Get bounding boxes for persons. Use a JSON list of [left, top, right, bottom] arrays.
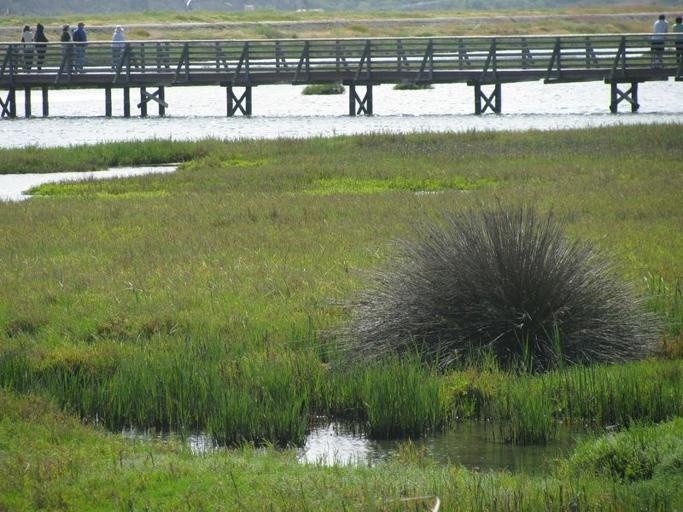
[[673, 16, 683, 67], [112, 25, 124, 69], [651, 15, 666, 69], [22, 24, 34, 71], [61, 24, 73, 72], [72, 23, 88, 72], [34, 23, 48, 71]]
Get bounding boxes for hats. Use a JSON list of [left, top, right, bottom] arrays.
[[24, 25, 30, 31], [114, 25, 124, 31], [62, 22, 86, 30], [37, 23, 44, 31]]
